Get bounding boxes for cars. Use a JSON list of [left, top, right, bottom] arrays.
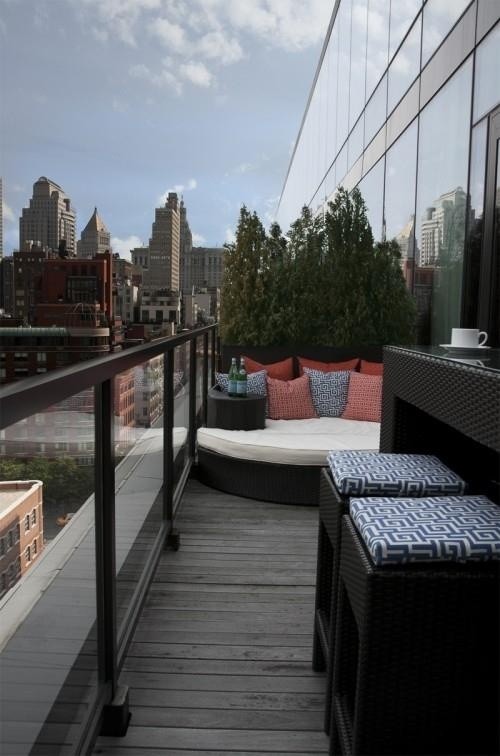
[[55, 510, 76, 526]]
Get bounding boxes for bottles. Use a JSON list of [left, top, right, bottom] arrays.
[[237, 356, 248, 397], [228, 357, 238, 396]]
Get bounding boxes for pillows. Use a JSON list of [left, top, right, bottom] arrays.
[[239, 355, 293, 382], [302, 367, 350, 417], [215, 370, 269, 417], [360, 360, 384, 375], [340, 371, 383, 422], [297, 357, 359, 378], [266, 374, 320, 419]]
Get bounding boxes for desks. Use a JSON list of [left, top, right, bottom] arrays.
[[207, 389, 266, 430], [380, 344, 500, 453]]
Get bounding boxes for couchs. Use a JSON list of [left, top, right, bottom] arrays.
[[194, 345, 383, 505]]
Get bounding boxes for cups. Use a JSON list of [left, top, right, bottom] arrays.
[[463, 359, 485, 367], [451, 327, 489, 346]]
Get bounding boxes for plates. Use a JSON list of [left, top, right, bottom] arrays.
[[441, 353, 492, 362], [438, 343, 492, 352]]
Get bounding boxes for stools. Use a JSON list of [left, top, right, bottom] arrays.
[[330, 494, 500, 756], [312, 449, 465, 735]]
